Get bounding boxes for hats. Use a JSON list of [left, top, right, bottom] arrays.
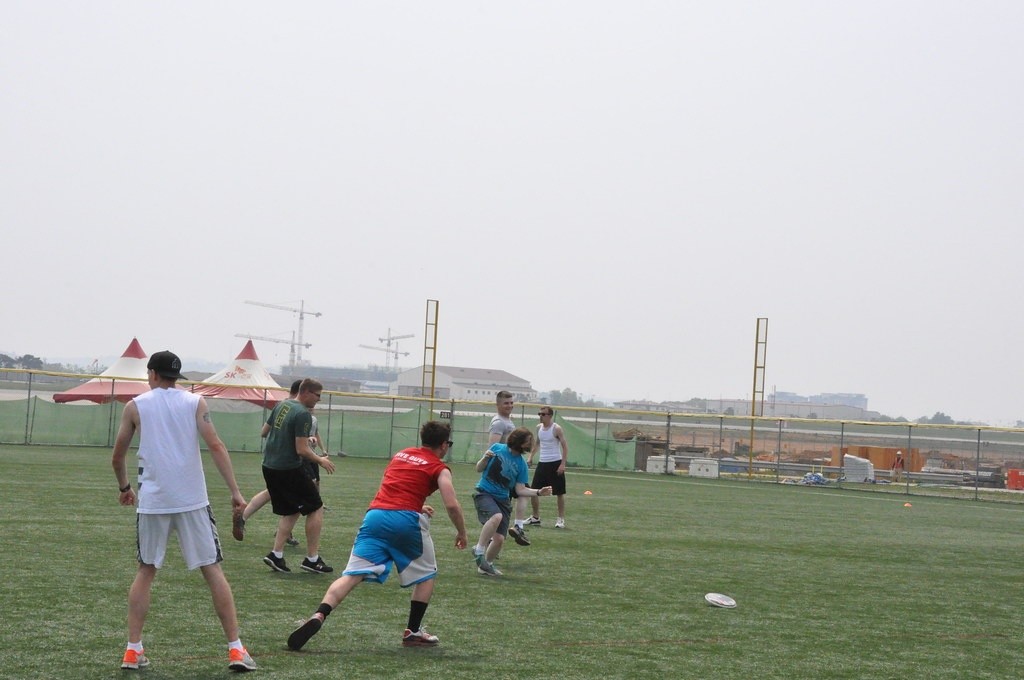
[[147, 351, 188, 381]]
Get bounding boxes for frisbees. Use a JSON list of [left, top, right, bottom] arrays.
[[705, 592, 737, 609]]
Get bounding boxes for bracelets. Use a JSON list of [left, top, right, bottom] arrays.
[[119, 482, 131, 492], [537, 489, 540, 496]]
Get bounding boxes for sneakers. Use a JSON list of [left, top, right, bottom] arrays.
[[555, 517, 564, 528], [228, 646, 256, 670], [478, 560, 503, 576], [287, 611, 324, 651], [300, 558, 333, 575], [471, 545, 486, 567], [509, 522, 530, 546], [523, 515, 540, 526], [487, 538, 500, 559], [121, 648, 149, 669], [273, 529, 300, 545], [402, 625, 439, 647], [263, 552, 291, 574], [233, 508, 246, 541]]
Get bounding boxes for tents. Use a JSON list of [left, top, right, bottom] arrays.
[[54, 337, 186, 403], [187, 340, 291, 411]]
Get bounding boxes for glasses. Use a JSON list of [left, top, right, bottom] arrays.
[[308, 391, 320, 398], [440, 440, 453, 447], [538, 413, 551, 416]]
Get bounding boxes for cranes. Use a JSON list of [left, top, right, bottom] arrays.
[[377, 326, 414, 376], [234, 329, 312, 375], [358, 341, 410, 381], [244, 298, 322, 366]]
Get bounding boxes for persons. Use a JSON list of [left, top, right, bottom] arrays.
[[523, 406, 568, 528], [112, 350, 257, 669], [892, 451, 905, 483], [310, 408, 331, 512], [233, 380, 299, 545], [472, 427, 554, 576], [288, 419, 467, 649], [488, 391, 532, 559], [261, 377, 336, 573]]
